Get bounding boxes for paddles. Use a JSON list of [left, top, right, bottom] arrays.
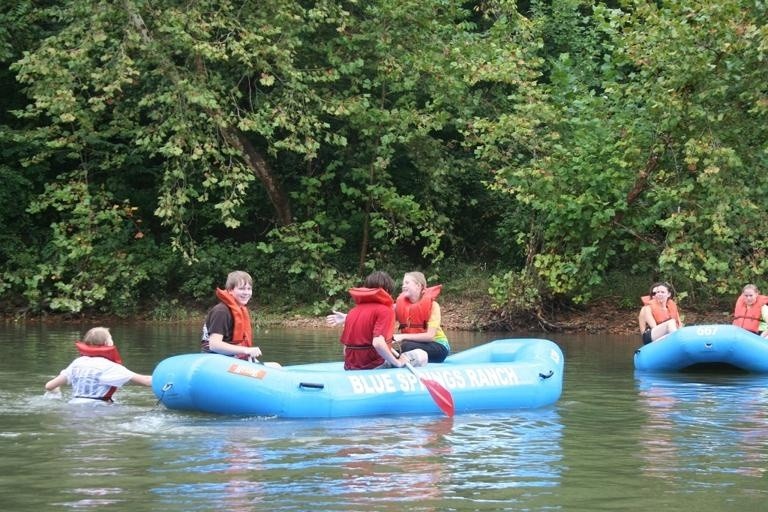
[[388, 347, 454, 420]]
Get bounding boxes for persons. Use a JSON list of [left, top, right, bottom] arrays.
[[45, 326, 153, 405], [642, 284, 681, 345], [732, 283, 768, 339], [328, 270, 450, 365], [638, 283, 659, 336], [339, 270, 429, 369], [200, 269, 282, 370]]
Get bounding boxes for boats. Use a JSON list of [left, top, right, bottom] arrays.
[[148, 337, 565, 423], [629, 321, 766, 378]]
